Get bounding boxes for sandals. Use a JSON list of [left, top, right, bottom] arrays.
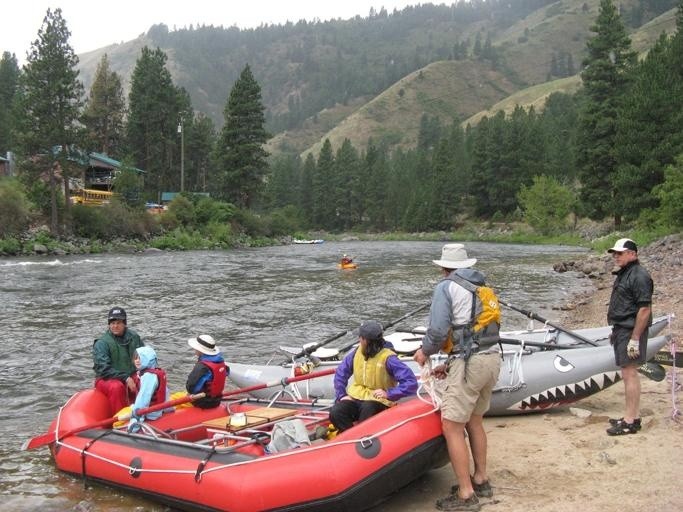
[[609, 417, 641, 427], [606, 424, 638, 436]]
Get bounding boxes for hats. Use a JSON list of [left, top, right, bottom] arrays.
[[188, 334, 221, 357], [107, 306, 126, 322], [608, 238, 640, 255], [433, 242, 478, 269], [358, 319, 383, 339]]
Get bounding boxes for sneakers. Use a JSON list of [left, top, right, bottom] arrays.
[[435, 493, 481, 512], [451, 475, 493, 499]]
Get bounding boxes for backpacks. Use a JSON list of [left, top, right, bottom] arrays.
[[269, 418, 311, 447], [470, 286, 504, 344]]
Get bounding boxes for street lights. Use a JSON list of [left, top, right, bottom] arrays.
[[176, 116, 186, 193]]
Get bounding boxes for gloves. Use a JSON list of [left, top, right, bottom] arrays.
[[626, 339, 640, 360]]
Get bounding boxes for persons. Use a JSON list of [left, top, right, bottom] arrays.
[[111, 346, 168, 436], [413, 243, 502, 510], [170, 334, 230, 407], [92, 307, 141, 417], [606, 237, 653, 436], [328, 322, 418, 435]]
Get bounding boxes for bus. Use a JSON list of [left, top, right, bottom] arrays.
[[72, 189, 116, 205]]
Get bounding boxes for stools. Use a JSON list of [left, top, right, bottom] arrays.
[[242, 406, 299, 421], [203, 412, 268, 438]]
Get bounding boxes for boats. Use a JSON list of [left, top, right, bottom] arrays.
[[46, 383, 456, 508], [383, 310, 674, 354], [241, 335, 674, 415], [295, 240, 315, 245], [337, 263, 356, 270], [314, 239, 324, 244]]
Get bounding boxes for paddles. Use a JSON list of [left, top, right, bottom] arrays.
[[19, 368, 206, 452], [498, 334, 683, 369], [498, 298, 666, 382]]
